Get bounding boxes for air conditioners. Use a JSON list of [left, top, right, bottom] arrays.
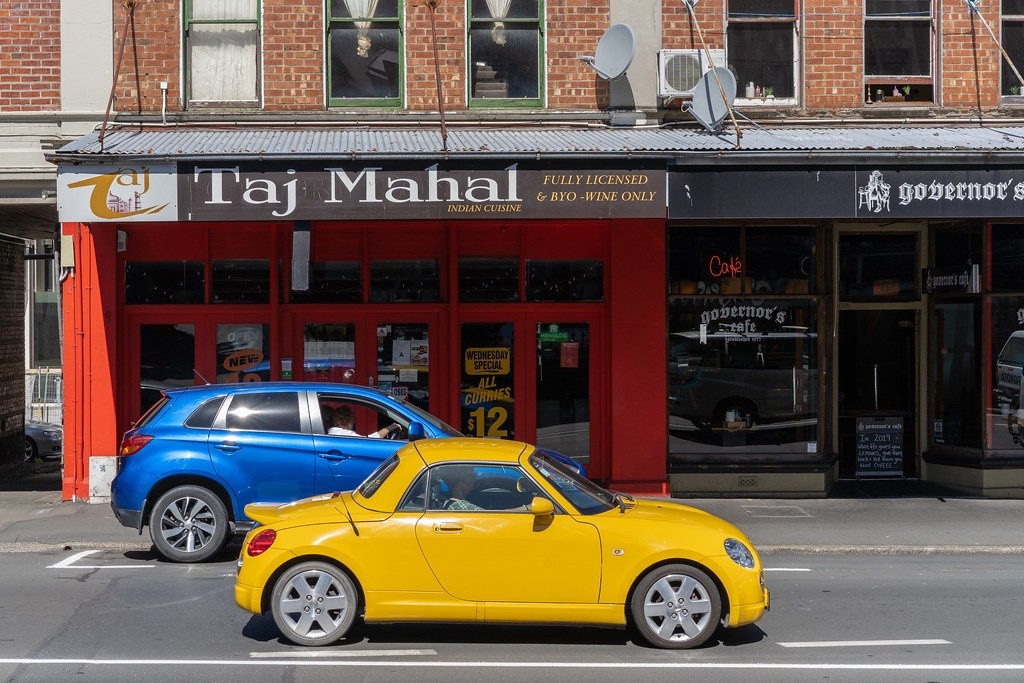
[[656, 48, 728, 107]]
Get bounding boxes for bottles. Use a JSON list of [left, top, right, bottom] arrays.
[[746, 414, 752, 428]]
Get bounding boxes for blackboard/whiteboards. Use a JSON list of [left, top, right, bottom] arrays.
[[855, 417, 904, 478]]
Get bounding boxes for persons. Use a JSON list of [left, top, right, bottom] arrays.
[[320, 404, 335, 435], [438, 464, 544, 512], [328, 404, 403, 439]]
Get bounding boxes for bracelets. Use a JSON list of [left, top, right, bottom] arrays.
[[523, 504, 529, 512]]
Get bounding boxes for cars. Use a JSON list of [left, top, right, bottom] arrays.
[[109, 382, 472, 563], [236, 437, 769, 648]]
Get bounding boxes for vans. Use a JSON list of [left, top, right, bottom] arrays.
[[672, 328, 822, 410], [995, 330, 1024, 421]]
